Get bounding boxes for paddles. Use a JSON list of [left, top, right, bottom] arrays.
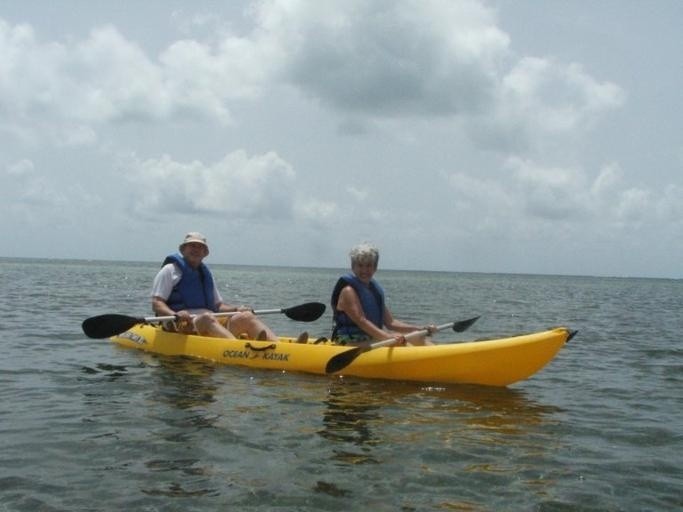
[[80, 302, 326, 339], [326, 315, 480, 374]]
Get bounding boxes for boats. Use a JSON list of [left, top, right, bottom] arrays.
[[107, 314, 576, 392]]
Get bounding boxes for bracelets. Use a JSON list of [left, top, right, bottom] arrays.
[[232, 306, 238, 312]]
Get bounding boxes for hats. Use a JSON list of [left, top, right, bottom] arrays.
[[180, 232, 209, 256]]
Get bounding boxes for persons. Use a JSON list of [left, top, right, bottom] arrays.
[[149, 231, 309, 346], [327, 243, 439, 349]]
